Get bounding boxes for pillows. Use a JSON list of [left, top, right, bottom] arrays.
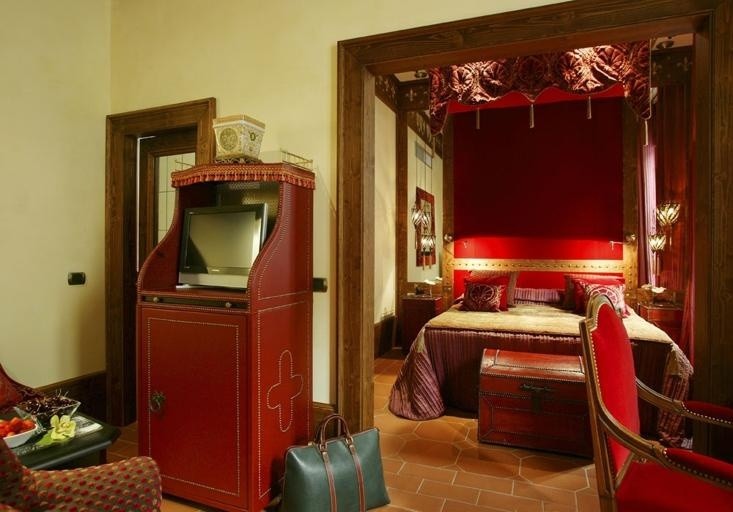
[[457, 269, 518, 313], [562, 272, 631, 318]]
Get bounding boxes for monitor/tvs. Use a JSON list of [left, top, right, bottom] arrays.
[[178, 203, 268, 290]]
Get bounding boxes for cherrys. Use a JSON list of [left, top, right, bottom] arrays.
[[17, 388, 78, 429]]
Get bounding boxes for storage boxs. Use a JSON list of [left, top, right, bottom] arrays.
[[477, 347, 594, 460]]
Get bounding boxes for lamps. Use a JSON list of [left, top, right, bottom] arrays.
[[648, 40, 681, 253], [411, 71, 436, 271], [443, 233, 456, 242], [610, 233, 636, 245]]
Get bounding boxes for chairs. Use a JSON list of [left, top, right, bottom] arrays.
[[0, 363, 46, 415], [579, 294, 733, 512], [0, 435, 163, 511]]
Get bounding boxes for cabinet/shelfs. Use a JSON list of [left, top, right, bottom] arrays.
[[136, 162, 315, 512]]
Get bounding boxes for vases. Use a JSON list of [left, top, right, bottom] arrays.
[[212, 116, 266, 164]]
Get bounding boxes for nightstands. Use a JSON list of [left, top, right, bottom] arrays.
[[401, 295, 444, 355], [636, 299, 683, 344]]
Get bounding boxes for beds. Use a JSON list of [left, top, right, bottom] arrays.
[[388, 288, 695, 451]]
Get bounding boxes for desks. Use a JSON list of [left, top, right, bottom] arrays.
[[0, 408, 121, 471]]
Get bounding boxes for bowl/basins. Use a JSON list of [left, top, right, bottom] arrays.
[[14, 396, 81, 431], [1, 423, 38, 450]]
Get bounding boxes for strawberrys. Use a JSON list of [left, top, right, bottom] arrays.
[[0, 416, 36, 438]]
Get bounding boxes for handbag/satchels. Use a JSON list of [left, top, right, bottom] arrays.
[[280, 411, 393, 512]]
